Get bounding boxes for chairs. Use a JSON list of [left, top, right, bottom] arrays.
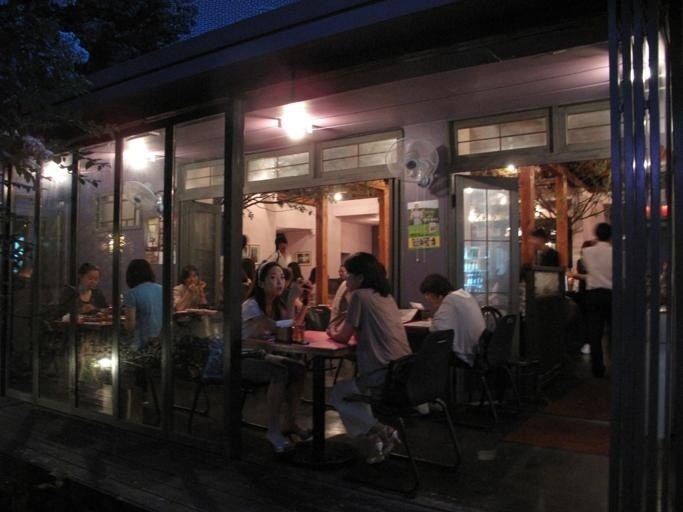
[[38, 290, 228, 421], [341, 328, 462, 484], [440, 313, 520, 426], [464, 307, 519, 415], [241, 374, 334, 440], [303, 305, 367, 390]]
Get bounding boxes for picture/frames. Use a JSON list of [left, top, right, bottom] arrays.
[[140, 215, 162, 251], [294, 250, 312, 267], [245, 242, 264, 266]]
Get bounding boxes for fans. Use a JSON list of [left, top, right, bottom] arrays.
[[382, 136, 443, 191], [120, 178, 167, 225]]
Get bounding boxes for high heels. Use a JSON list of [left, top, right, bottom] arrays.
[[266, 429, 292, 452], [280, 425, 314, 442], [366, 425, 400, 464]]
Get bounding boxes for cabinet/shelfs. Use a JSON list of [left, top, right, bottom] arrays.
[[522, 260, 574, 406]]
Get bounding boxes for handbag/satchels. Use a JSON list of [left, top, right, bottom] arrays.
[[305, 308, 330, 331]]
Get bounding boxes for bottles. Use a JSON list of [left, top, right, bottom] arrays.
[[463, 246, 506, 287]]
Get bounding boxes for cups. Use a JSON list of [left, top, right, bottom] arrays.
[[292, 322, 305, 342], [276, 326, 291, 343]]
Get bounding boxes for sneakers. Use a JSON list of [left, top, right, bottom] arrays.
[[430, 398, 446, 411], [413, 402, 429, 414]]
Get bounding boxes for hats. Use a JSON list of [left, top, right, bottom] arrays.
[[275, 233, 287, 245]]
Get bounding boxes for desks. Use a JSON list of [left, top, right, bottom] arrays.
[[243, 329, 362, 473], [403, 321, 437, 354]]
